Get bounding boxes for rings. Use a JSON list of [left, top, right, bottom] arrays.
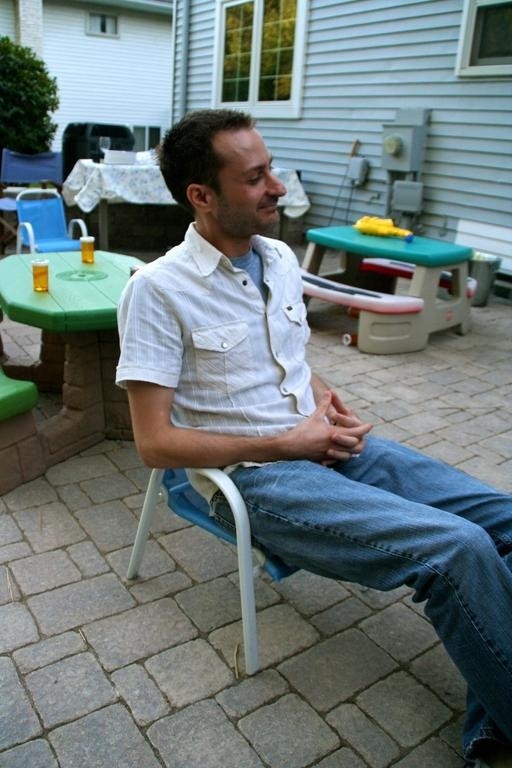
[[350, 453, 361, 460]]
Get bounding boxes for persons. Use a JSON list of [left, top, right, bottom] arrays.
[[115, 110, 512, 768]]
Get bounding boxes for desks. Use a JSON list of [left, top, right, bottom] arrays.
[[0, 251, 149, 460], [301, 225, 478, 351], [60, 156, 312, 255]]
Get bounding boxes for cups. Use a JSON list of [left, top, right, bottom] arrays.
[[32, 260, 48, 292], [80, 236, 95, 264]]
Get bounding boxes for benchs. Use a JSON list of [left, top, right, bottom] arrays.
[[299, 268, 423, 352], [363, 258, 479, 324]]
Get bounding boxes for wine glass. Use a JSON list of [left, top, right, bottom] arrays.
[[99, 136, 111, 162]]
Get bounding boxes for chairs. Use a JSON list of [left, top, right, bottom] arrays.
[[0, 149, 65, 250], [128, 450, 306, 680], [16, 188, 87, 256]]
